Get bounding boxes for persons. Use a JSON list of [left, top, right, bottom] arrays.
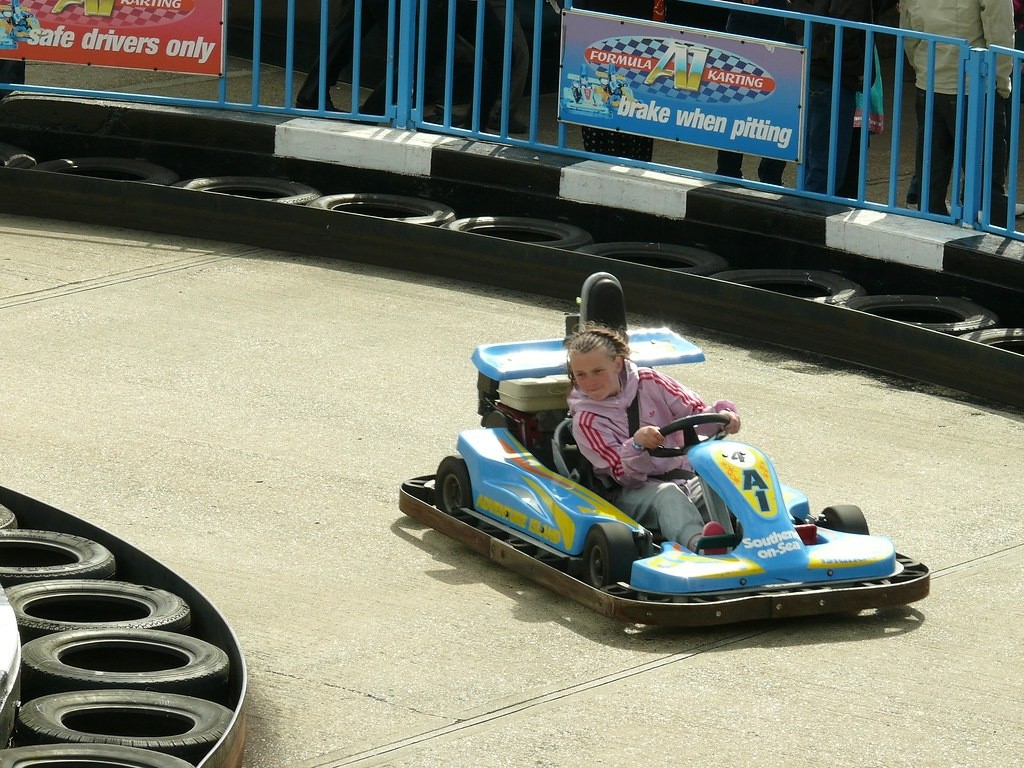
[[708, 0, 797, 186], [295, 0, 529, 135], [899, 0, 1024, 228], [581, 0, 654, 162], [784, 0, 915, 199], [567, 325, 740, 555]]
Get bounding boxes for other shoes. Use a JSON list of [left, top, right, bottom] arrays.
[[702, 522, 727, 556], [296, 75, 351, 121], [1014, 203, 1024, 216], [434, 105, 468, 127], [488, 114, 527, 134], [906, 199, 950, 211], [946, 205, 983, 228]]
